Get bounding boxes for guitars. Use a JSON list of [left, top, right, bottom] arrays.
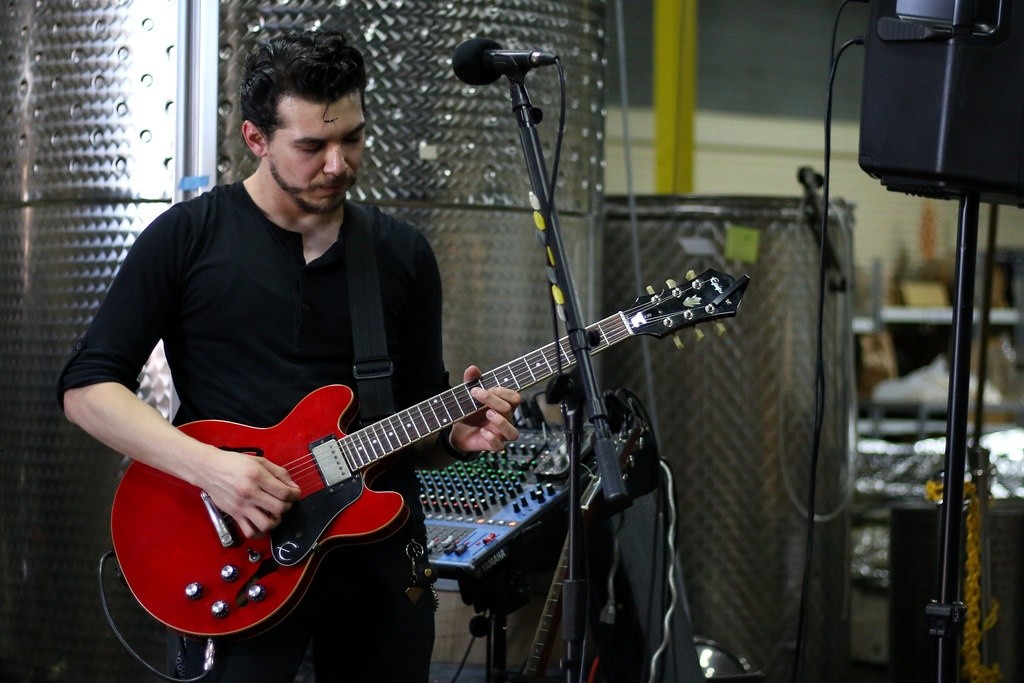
[[111, 266, 750, 644]]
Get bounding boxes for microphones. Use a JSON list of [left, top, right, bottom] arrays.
[[453, 39, 560, 86]]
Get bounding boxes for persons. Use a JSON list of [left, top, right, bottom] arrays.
[[57, 32, 522, 682]]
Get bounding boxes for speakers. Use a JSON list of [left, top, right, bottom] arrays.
[[858, 2, 1023, 204]]
